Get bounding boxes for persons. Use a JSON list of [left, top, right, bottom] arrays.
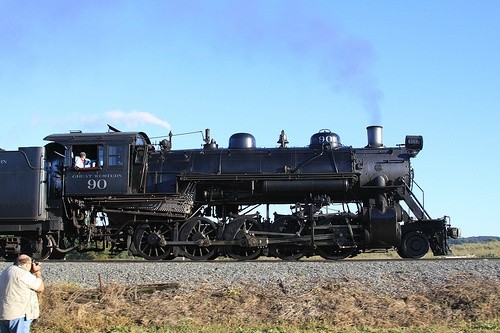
[[0, 254, 44, 333], [75, 152, 86, 168]]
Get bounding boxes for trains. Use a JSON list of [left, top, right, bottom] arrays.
[[0, 125, 461, 262]]
